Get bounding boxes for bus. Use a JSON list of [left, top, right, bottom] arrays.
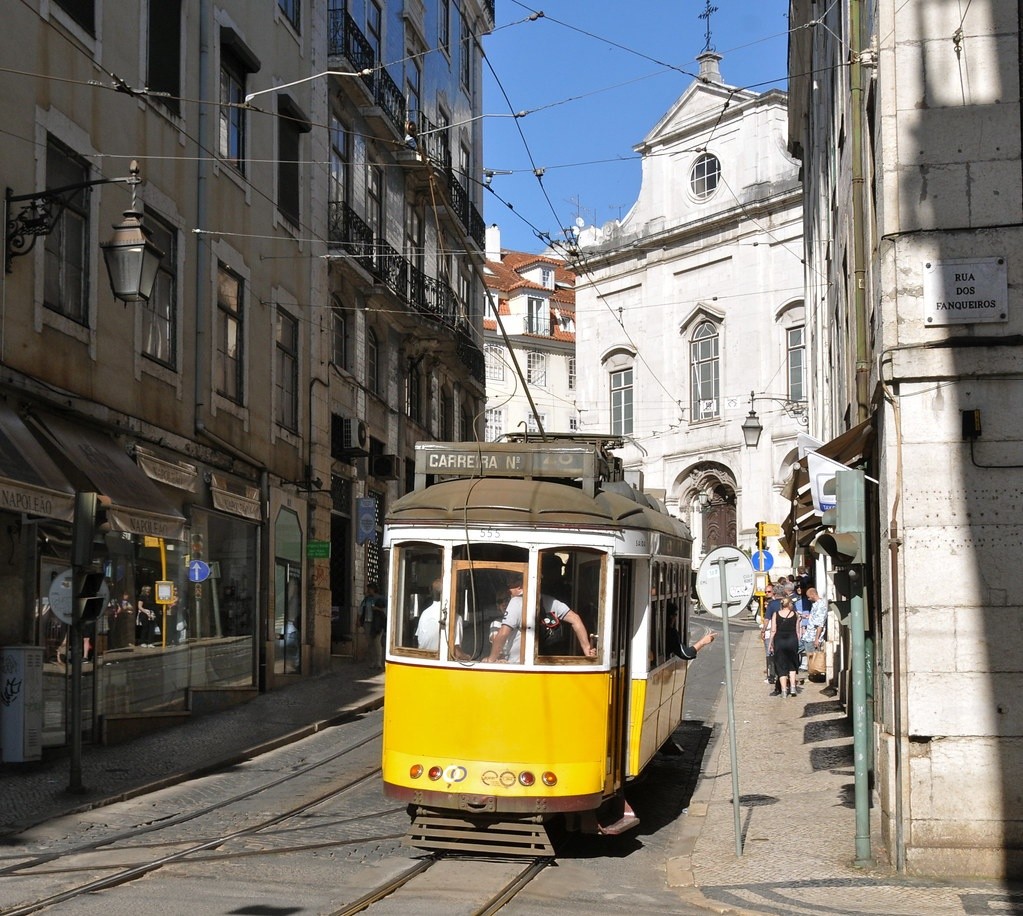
[[380, 120, 696, 859]]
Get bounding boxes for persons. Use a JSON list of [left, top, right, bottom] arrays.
[[414, 551, 597, 662], [357, 583, 387, 668], [293, 613, 301, 668], [666, 603, 718, 660], [53, 623, 94, 664], [754, 566, 827, 698], [108, 582, 189, 649]]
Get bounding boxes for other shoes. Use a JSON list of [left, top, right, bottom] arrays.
[[781, 691, 789, 699], [148, 644, 155, 648], [140, 643, 147, 648], [764, 679, 768, 683], [791, 687, 798, 696]]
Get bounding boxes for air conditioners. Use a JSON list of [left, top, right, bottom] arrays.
[[342, 419, 372, 452], [375, 454, 401, 481]]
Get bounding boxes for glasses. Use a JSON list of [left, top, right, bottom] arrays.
[[670, 613, 677, 617], [765, 591, 771, 593], [497, 596, 510, 604]]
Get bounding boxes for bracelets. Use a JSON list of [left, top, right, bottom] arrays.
[[761, 631, 765, 633]]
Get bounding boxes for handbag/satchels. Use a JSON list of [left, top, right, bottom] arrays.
[[766, 652, 778, 684], [808, 648, 826, 675]]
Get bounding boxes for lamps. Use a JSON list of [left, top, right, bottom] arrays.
[[741, 390, 808, 449], [4, 159, 165, 308]]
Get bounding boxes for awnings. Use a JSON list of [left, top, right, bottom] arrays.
[[0, 397, 76, 522], [776, 416, 873, 569], [126, 443, 198, 495], [23, 401, 188, 543], [205, 472, 262, 521]]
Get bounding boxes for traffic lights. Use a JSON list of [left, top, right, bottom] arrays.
[[821, 471, 864, 527], [79, 597, 105, 622], [76, 570, 106, 596], [755, 521, 767, 549], [68, 491, 113, 567], [814, 532, 867, 566]]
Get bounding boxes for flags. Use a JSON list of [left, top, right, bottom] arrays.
[[806, 452, 851, 516]]
